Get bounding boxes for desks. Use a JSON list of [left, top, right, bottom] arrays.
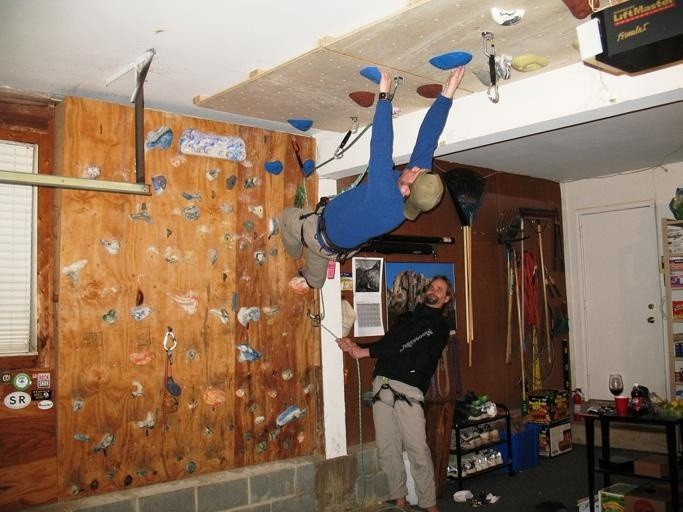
[[579, 399, 683, 512]]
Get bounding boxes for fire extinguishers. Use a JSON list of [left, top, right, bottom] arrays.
[[571, 388, 582, 421]]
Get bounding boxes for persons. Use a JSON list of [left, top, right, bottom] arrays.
[[278, 65, 466, 287], [333, 276, 452, 512]]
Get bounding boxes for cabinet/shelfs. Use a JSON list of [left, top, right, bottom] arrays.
[[447, 403, 514, 491], [661, 218, 683, 401]]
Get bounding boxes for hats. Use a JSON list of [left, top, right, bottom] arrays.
[[403, 170, 445, 222]]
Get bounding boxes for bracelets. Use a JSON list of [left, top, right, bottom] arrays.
[[379, 91, 395, 102]]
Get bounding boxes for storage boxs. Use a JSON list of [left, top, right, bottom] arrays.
[[528, 388, 572, 457], [598, 483, 639, 512], [624, 480, 673, 512]]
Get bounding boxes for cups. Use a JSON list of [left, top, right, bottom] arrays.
[[609, 375, 629, 417]]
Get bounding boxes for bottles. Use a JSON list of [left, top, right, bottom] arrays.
[[631, 383, 643, 417]]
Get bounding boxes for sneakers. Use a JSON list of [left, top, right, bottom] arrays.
[[447, 399, 504, 480]]
